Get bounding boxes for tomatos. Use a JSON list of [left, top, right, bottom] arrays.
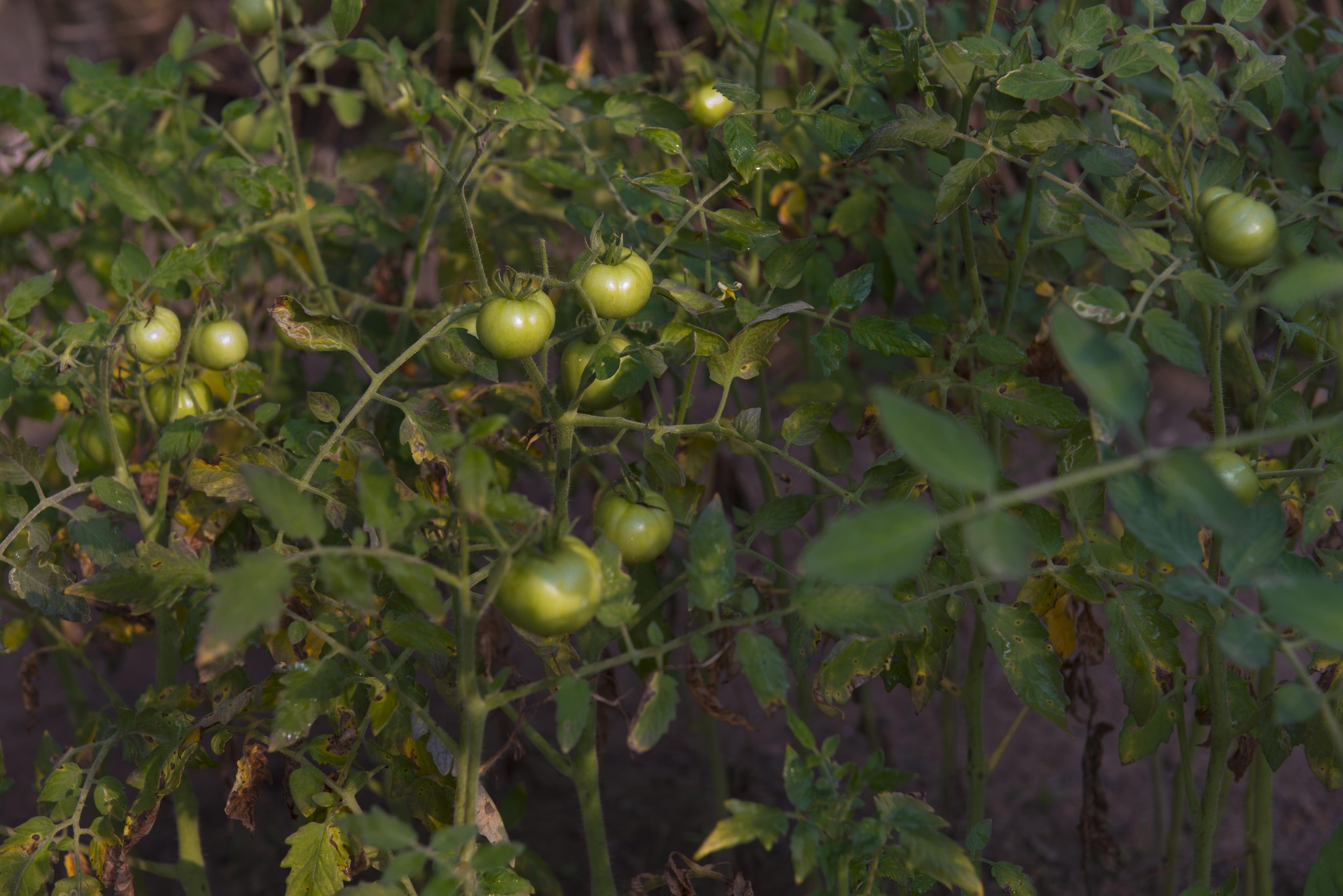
[[0, 83, 1277, 634]]
